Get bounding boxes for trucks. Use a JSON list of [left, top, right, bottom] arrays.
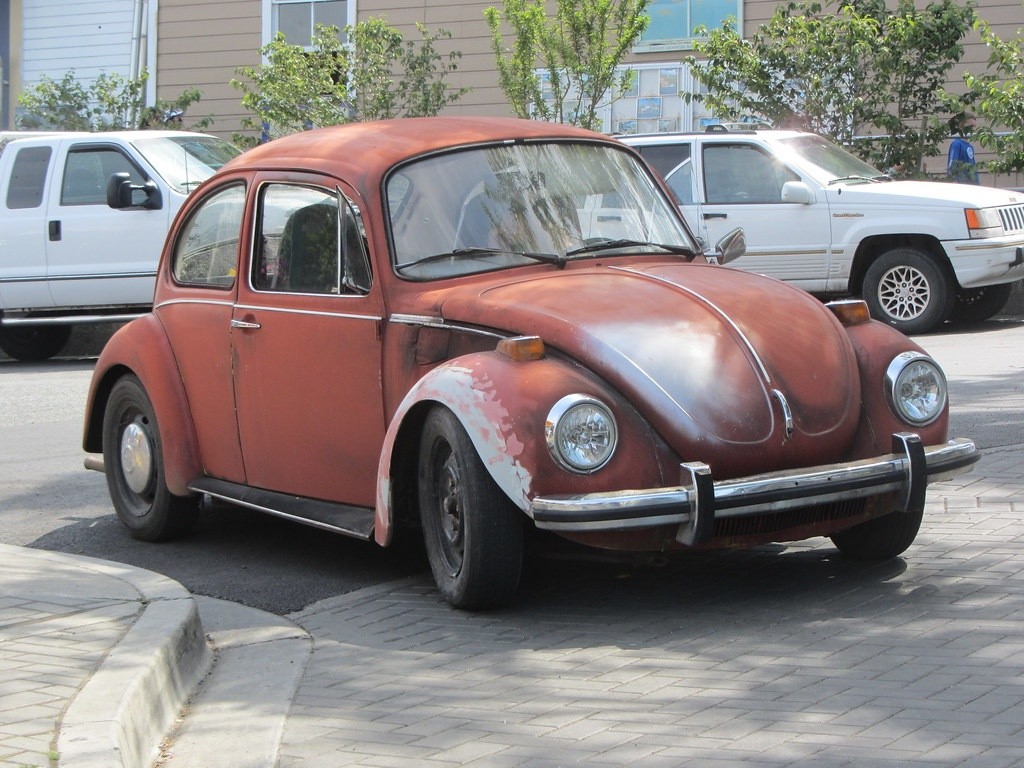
[[0, 130, 344, 364]]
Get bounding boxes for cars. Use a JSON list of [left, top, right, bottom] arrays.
[[81, 113, 983, 610]]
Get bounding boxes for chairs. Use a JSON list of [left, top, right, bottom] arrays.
[[69, 169, 100, 198], [457, 191, 523, 249], [270, 204, 370, 294]]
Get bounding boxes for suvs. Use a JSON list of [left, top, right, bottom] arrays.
[[570, 128, 1024, 334]]
